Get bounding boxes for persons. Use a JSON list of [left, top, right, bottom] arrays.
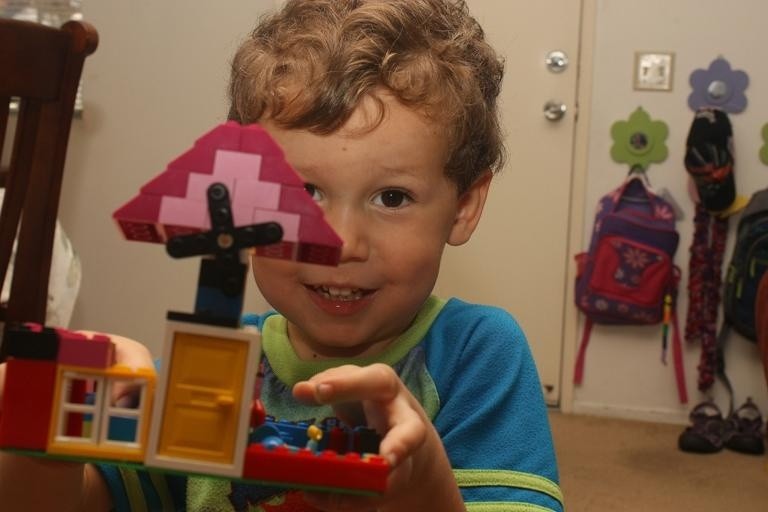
[[1, 0, 566, 512]]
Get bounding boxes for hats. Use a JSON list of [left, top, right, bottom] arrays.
[[685, 108, 736, 210]]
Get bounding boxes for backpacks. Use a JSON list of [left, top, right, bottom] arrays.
[[715, 184, 768, 399], [574, 162, 687, 406]]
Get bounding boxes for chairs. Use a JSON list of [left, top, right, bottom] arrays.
[[0, 18, 107, 332]]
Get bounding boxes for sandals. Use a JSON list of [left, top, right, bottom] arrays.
[[677, 399, 766, 454]]
